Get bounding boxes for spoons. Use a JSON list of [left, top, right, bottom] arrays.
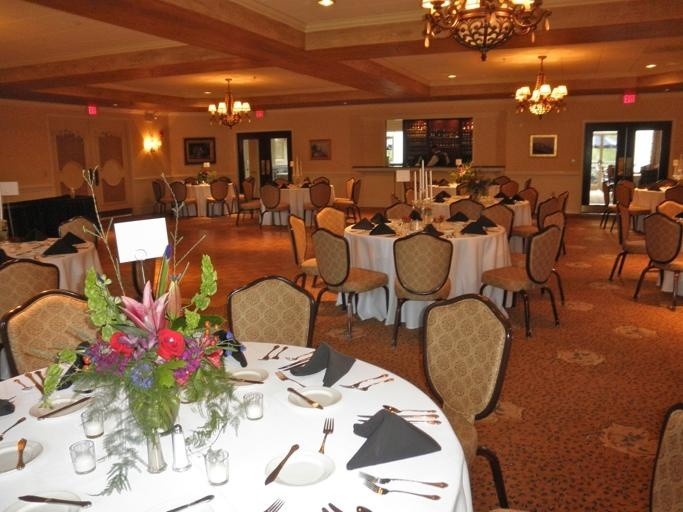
[[356, 505, 372, 511], [17, 438, 26, 469], [383, 405, 438, 413], [12, 379, 30, 390]]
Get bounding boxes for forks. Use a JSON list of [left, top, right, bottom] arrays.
[[259, 345, 288, 360], [274, 370, 304, 387], [359, 473, 446, 500], [286, 351, 314, 361], [339, 374, 393, 391], [317, 417, 334, 453], [263, 498, 286, 512]]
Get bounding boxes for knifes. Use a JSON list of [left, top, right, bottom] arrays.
[[166, 494, 214, 511], [357, 414, 441, 425], [263, 444, 299, 485], [17, 495, 91, 507], [321, 502, 344, 512], [0, 416, 25, 441], [286, 388, 324, 410], [24, 370, 46, 394], [227, 377, 265, 384]]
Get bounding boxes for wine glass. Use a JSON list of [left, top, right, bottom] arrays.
[[455, 159, 461, 168]]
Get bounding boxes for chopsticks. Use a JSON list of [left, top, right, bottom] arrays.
[[277, 357, 309, 370], [37, 397, 91, 419]]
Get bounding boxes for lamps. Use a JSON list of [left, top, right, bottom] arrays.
[[420, 0, 552, 61], [142, 129, 162, 153], [514, 56, 569, 120], [207, 78, 253, 130]]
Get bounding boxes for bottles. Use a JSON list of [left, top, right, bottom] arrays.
[[172, 424, 191, 471]]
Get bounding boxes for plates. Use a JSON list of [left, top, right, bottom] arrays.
[[0, 439, 43, 474], [0, 399, 14, 416], [226, 368, 269, 386], [349, 212, 501, 238], [29, 398, 85, 419], [0, 491, 82, 512], [70, 243, 90, 249], [287, 385, 342, 408], [0, 238, 61, 259], [265, 451, 333, 485]]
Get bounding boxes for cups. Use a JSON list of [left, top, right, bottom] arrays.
[[204, 450, 229, 485], [68, 440, 96, 475], [242, 391, 263, 420], [80, 408, 103, 437]]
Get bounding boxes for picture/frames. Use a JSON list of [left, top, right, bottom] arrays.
[[184, 137, 216, 165], [529, 134, 558, 158], [309, 139, 331, 161]]
